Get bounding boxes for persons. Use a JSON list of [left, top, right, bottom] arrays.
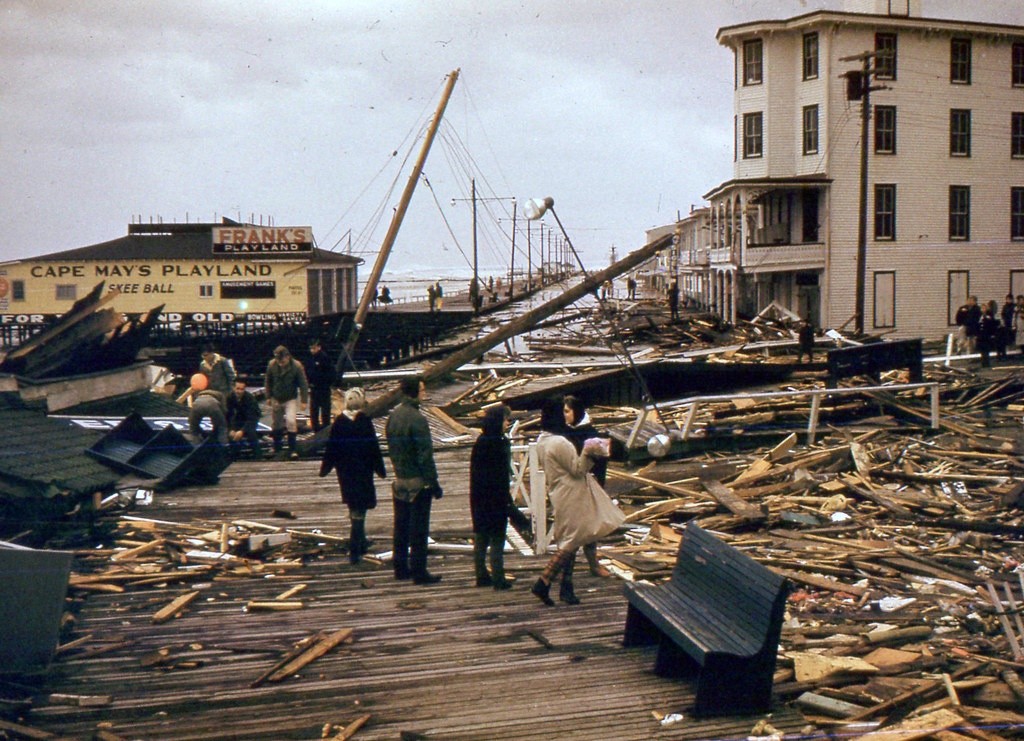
[[427, 282, 443, 312], [266, 345, 308, 460], [469, 406, 512, 589], [371, 285, 393, 311], [667, 283, 679, 321], [489, 276, 502, 294], [602, 279, 614, 300], [467, 279, 479, 302], [956, 294, 1024, 368], [626, 277, 636, 300], [531, 394, 627, 607], [189, 342, 264, 463], [385, 376, 443, 585], [795, 319, 814, 365], [305, 339, 336, 433], [318, 388, 387, 563]]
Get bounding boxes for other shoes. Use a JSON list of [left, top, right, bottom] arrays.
[[559, 589, 580, 604], [476, 576, 493, 587], [494, 580, 512, 590], [590, 565, 610, 578], [531, 577, 555, 606], [414, 574, 442, 585], [396, 571, 412, 580]]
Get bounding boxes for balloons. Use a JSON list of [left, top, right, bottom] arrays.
[[190, 373, 208, 391]]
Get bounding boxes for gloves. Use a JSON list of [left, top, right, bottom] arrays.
[[432, 485, 442, 499]]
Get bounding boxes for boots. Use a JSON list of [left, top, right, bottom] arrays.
[[351, 517, 368, 564], [272, 428, 299, 458]]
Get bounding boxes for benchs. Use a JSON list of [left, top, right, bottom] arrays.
[[85, 413, 234, 493], [489, 291, 498, 303], [814, 337, 925, 389], [623, 523, 796, 719]]
